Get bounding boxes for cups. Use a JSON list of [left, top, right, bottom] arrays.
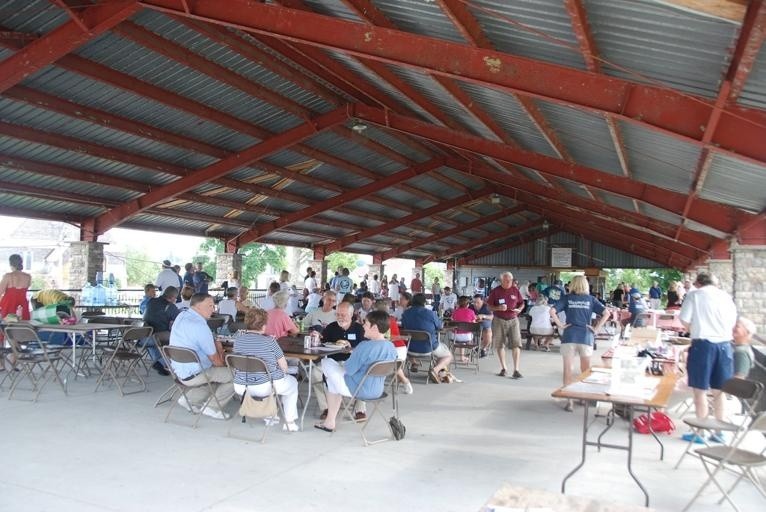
[[312, 337, 321, 347]]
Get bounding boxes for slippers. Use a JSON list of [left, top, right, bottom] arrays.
[[314, 424, 332, 432]]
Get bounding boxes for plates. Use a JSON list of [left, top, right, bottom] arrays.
[[323, 341, 348, 347]]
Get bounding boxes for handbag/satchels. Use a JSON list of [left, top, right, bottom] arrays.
[[238, 390, 276, 418], [633, 412, 675, 433]]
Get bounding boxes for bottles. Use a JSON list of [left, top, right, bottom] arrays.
[[300, 320, 304, 332]]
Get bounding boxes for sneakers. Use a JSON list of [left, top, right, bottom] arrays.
[[355, 412, 366, 422], [512, 372, 521, 378], [282, 423, 298, 432], [403, 378, 412, 395], [319, 409, 327, 419], [499, 370, 508, 376], [428, 369, 440, 384], [682, 433, 724, 444], [152, 360, 169, 375], [177, 395, 229, 419]]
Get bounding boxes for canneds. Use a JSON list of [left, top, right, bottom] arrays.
[[311, 336, 320, 346]]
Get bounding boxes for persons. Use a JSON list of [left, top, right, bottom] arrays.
[[623, 292, 642, 328], [0, 254, 32, 371], [529, 294, 554, 351], [449, 296, 476, 360], [140, 284, 155, 314], [550, 275, 609, 409], [678, 271, 737, 444], [402, 294, 453, 383], [649, 281, 661, 309], [312, 303, 368, 421], [31, 289, 75, 310], [732, 316, 757, 380], [613, 282, 642, 307], [315, 312, 397, 430], [219, 286, 246, 336], [235, 309, 299, 432], [487, 272, 524, 378], [264, 291, 298, 337], [143, 286, 179, 375], [469, 294, 492, 356], [528, 276, 571, 304], [667, 280, 681, 308], [270, 265, 376, 327], [156, 260, 213, 309], [169, 292, 236, 419], [239, 287, 256, 309], [381, 274, 457, 318]]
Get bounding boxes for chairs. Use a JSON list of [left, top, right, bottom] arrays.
[[0, 315, 480, 446], [514, 304, 766, 512]]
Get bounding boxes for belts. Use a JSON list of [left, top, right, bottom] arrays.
[[182, 372, 199, 381]]
